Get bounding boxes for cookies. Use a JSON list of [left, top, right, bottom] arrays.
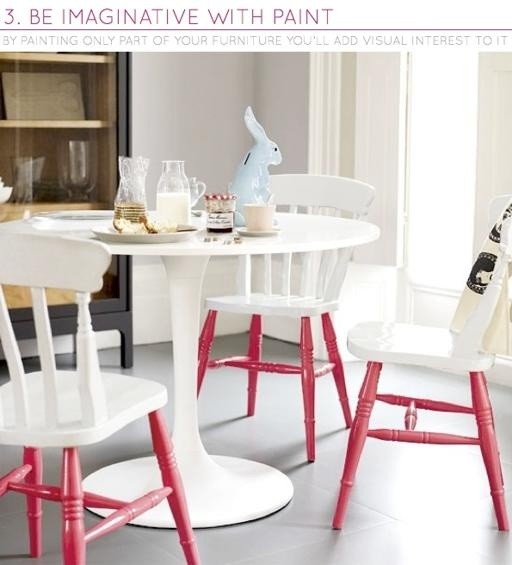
[[113, 204, 147, 233]]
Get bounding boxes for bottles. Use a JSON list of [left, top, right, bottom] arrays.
[[156, 160, 206, 230], [113, 155, 150, 232]]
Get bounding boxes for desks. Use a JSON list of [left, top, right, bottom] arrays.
[[2, 209, 381, 530]]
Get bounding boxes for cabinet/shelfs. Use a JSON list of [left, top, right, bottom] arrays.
[[0, 51, 133, 369]]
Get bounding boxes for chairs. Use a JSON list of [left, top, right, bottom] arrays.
[[0, 227, 200, 564], [190, 172, 510, 534]]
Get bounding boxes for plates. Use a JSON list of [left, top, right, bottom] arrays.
[[237, 230, 278, 237], [92, 223, 205, 244]]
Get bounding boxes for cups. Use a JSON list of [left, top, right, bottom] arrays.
[[244, 205, 276, 232], [10, 156, 46, 203]]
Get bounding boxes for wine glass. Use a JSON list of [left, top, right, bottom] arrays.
[[55, 138, 99, 202]]
